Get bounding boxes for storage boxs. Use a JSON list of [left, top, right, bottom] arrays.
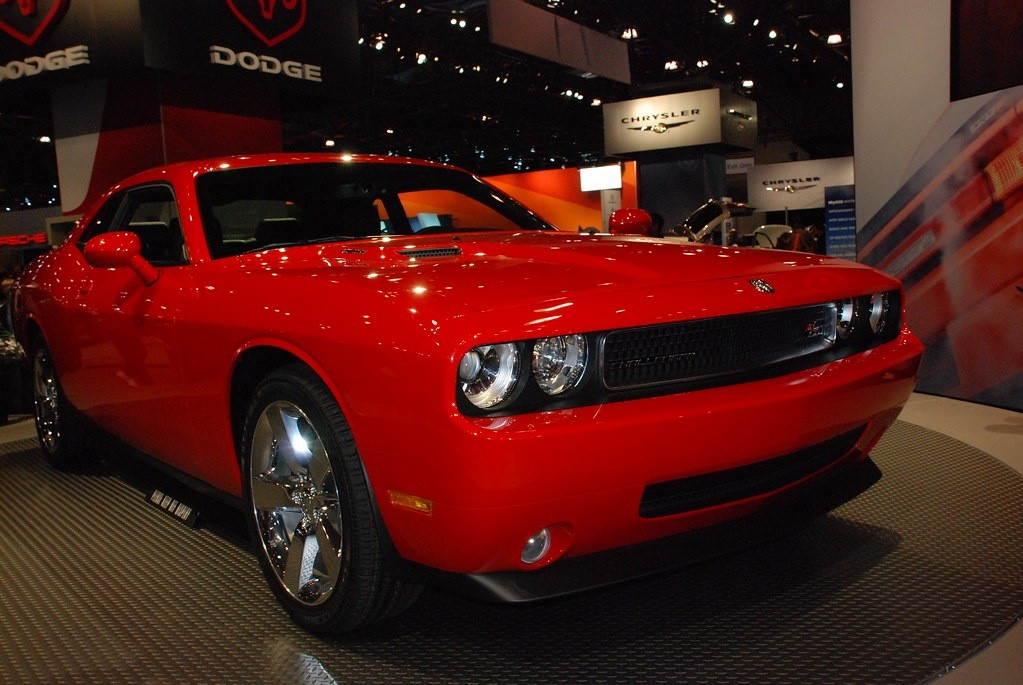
[[45, 214, 82, 245]]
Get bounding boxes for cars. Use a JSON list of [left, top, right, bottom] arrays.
[[660, 194, 824, 254]]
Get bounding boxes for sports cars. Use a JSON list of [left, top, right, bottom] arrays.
[[10, 149, 928, 640]]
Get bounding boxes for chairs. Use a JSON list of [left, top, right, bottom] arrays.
[[168, 216, 225, 262], [128, 221, 168, 235], [254, 218, 297, 238]]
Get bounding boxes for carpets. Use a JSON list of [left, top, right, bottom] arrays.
[[0, 420, 1023, 685]]
